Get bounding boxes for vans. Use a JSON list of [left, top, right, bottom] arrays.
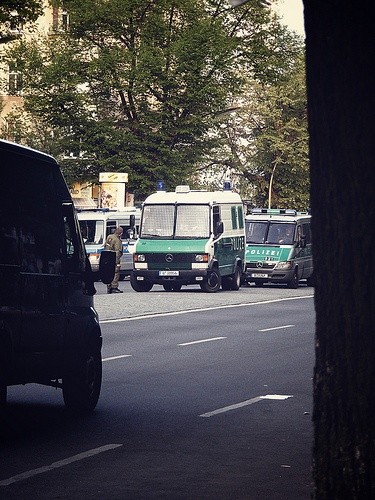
[[240, 209, 314, 290], [130, 180, 246, 294], [73, 206, 142, 283], [0, 137, 104, 415]]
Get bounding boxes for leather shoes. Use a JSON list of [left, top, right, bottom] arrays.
[[106, 287, 123, 293]]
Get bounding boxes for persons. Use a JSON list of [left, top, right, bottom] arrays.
[[104, 227, 124, 294]]
